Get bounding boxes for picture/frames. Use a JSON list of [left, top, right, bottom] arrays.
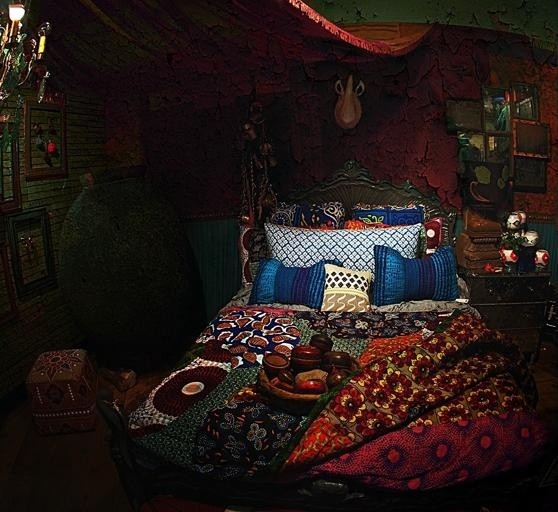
[[0, 97, 67, 325], [445, 82, 551, 194]]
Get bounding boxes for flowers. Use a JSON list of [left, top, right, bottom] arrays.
[[494, 232, 528, 250]]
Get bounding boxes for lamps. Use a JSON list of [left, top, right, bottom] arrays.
[[0, 1, 54, 122]]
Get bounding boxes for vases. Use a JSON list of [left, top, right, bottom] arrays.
[[498, 247, 520, 271]]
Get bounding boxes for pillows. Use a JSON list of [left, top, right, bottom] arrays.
[[248, 213, 460, 314]]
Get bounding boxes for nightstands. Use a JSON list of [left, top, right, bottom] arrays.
[[458, 268, 552, 375]]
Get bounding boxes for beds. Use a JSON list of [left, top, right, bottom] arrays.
[[111, 160, 556, 512]]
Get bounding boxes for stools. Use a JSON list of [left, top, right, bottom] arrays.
[[26, 348, 99, 433]]
